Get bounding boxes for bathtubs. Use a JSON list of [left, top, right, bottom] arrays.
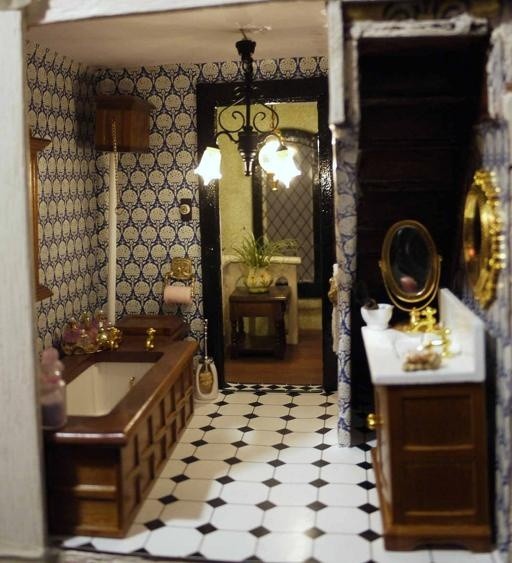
[[26, 335, 198, 539]]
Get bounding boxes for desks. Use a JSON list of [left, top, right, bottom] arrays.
[[228, 285, 293, 359]]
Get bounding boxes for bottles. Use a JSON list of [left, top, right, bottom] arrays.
[[40, 347, 67, 432], [62, 309, 122, 356]]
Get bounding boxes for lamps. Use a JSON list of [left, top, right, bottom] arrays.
[[193, 27, 303, 191]]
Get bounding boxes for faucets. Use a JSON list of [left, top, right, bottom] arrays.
[[145, 327, 157, 350], [417, 322, 451, 356], [409, 307, 437, 333]]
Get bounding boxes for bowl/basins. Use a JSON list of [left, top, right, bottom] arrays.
[[359, 303, 394, 332]]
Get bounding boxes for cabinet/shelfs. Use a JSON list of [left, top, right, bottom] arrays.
[[366, 379, 496, 551]]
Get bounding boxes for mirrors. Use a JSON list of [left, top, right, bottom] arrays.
[[460, 169, 504, 300], [375, 218, 443, 334]]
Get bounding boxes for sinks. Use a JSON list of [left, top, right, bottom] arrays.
[[388, 331, 438, 366]]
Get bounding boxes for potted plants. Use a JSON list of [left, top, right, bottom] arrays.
[[234, 232, 302, 293]]
